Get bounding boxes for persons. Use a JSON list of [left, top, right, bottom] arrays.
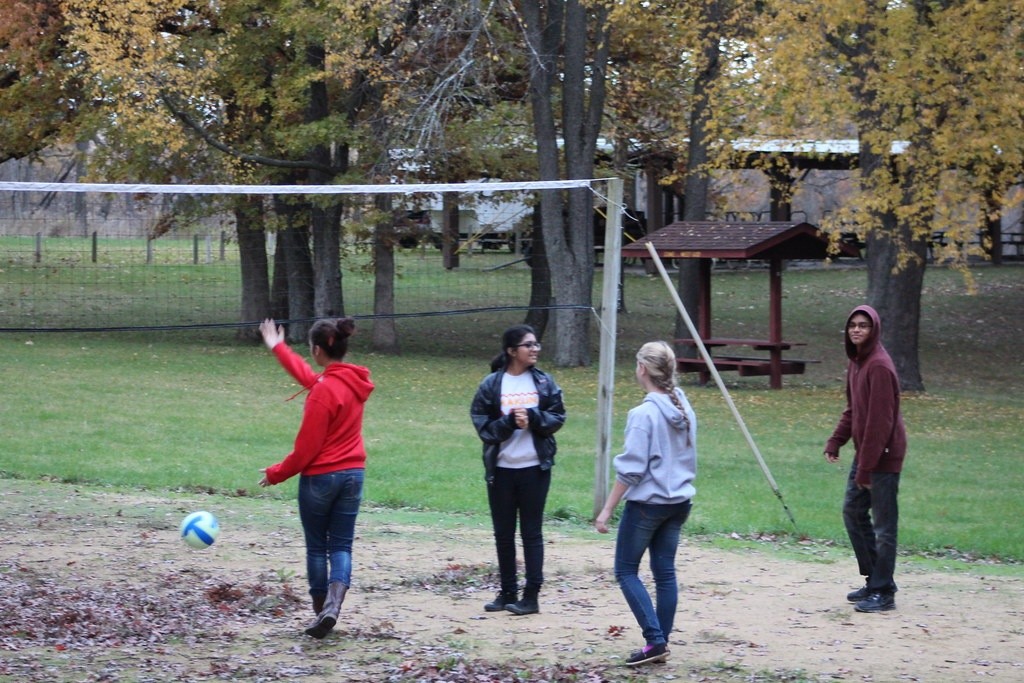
[[469, 324, 566, 615], [595, 341, 697, 665], [822, 304, 907, 612], [258, 317, 375, 639]]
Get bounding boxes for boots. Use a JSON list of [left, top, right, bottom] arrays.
[[305, 582, 348, 639], [310, 592, 327, 619]]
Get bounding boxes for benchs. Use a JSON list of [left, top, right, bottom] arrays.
[[718, 355, 822, 363], [676, 358, 770, 367]]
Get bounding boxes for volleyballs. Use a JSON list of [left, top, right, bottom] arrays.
[[179, 510, 219, 550]]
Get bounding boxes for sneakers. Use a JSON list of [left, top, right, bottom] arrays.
[[503, 594, 539, 615], [855, 590, 895, 613], [847, 585, 873, 601], [485, 590, 517, 611]]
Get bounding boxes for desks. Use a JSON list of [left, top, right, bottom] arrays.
[[671, 337, 809, 389]]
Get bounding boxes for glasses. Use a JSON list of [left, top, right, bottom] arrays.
[[850, 323, 873, 329], [510, 342, 542, 348]]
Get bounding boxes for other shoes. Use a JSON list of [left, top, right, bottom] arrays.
[[625, 643, 670, 666]]
